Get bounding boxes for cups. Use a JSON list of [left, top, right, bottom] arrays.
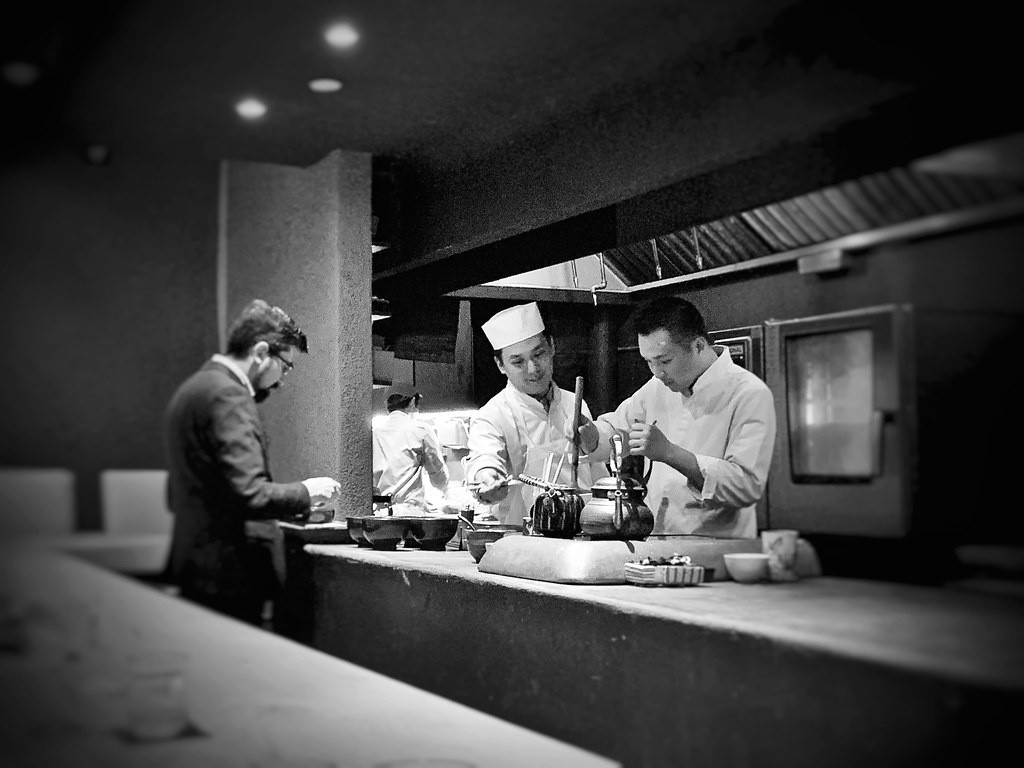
[[761, 529, 798, 582]]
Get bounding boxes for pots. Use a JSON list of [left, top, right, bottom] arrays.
[[467, 521, 524, 531]]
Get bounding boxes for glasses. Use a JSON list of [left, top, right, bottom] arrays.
[[268, 347, 294, 376]]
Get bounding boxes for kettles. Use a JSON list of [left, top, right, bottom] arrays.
[[579, 433, 654, 541]]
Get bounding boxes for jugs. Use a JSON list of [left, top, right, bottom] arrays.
[[518, 473, 585, 534]]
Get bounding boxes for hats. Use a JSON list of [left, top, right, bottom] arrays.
[[480, 301, 544, 351], [387, 382, 422, 409]]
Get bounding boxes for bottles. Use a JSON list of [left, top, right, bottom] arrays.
[[461, 505, 474, 550]]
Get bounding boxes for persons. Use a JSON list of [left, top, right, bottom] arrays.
[[567, 295, 776, 539], [369, 391, 451, 517], [160, 297, 343, 633], [462, 301, 613, 531]]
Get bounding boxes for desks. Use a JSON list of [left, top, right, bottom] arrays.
[[0, 530, 624, 767], [302, 534, 1024, 768]]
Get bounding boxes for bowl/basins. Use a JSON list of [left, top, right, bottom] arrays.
[[465, 530, 506, 563], [723, 553, 771, 580], [345, 515, 383, 548], [361, 516, 410, 550], [399, 516, 437, 548], [405, 519, 459, 551]]
